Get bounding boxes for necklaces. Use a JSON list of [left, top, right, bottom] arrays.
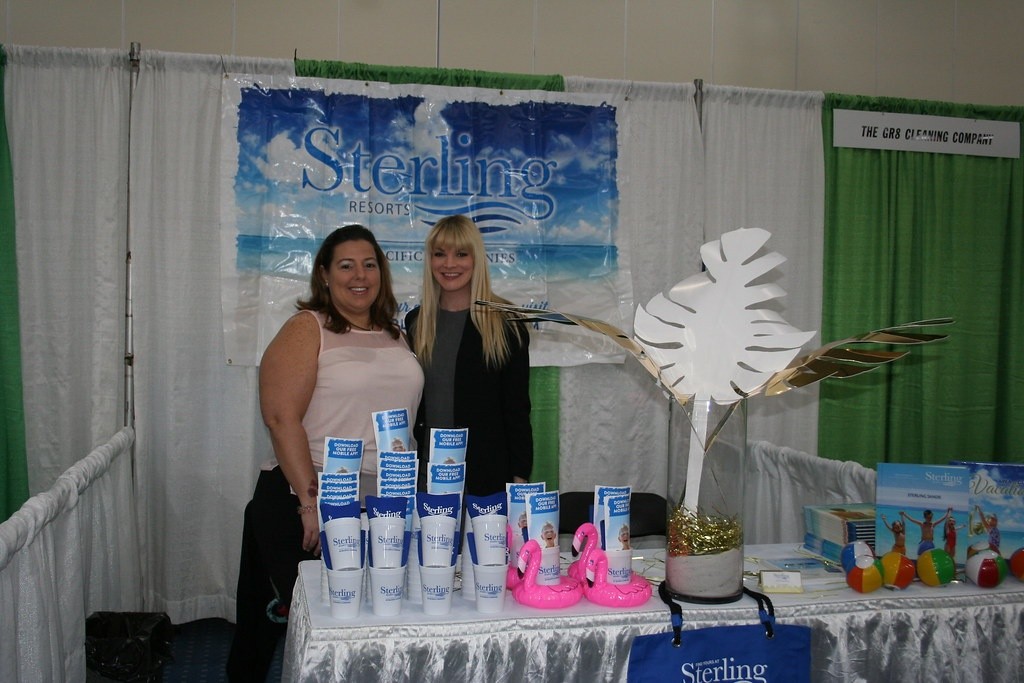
[[348, 321, 374, 331]]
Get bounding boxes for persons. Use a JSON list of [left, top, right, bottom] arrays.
[[942, 508, 966, 557], [406, 215, 533, 496], [618, 524, 629, 549], [225, 225, 426, 683], [341, 437, 558, 548], [903, 508, 950, 555], [880, 511, 906, 556], [975, 503, 1000, 547]]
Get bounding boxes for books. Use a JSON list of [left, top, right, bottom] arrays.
[[761, 462, 1024, 584]]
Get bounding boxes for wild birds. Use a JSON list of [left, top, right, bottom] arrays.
[[505, 522, 652, 610]]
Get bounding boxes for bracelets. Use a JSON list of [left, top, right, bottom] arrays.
[[299, 504, 317, 513]]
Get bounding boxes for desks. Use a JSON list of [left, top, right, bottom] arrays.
[[279, 541, 1024, 683]]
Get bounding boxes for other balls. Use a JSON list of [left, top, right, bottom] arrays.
[[1010, 548, 1024, 582], [845, 554, 885, 594], [880, 551, 915, 590], [840, 540, 875, 571], [966, 541, 1002, 559], [917, 548, 955, 587], [965, 550, 1007, 589]]
